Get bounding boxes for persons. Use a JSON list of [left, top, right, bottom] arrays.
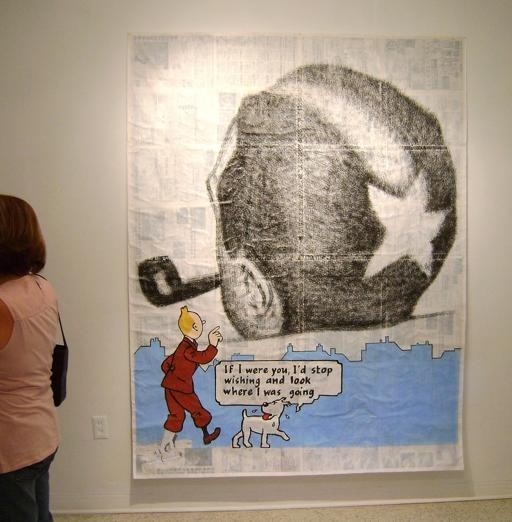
[[153, 305, 224, 460], [0, 193, 60, 522], [206, 59, 460, 340]]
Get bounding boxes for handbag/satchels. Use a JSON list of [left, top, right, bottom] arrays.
[[50, 343, 68, 406]]
